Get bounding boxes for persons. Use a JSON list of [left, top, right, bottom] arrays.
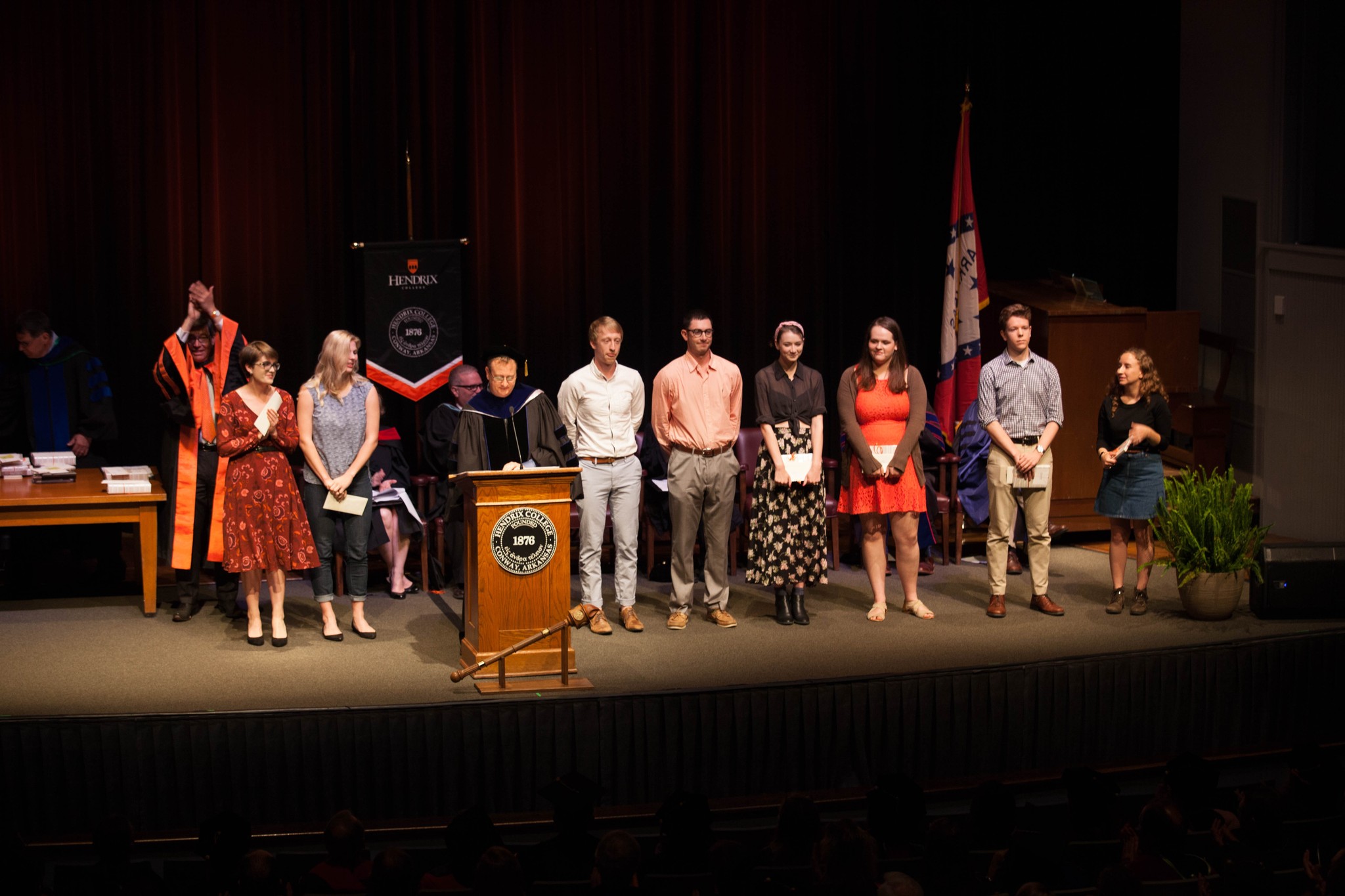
[[218, 341, 320, 647], [831, 395, 1031, 575], [364, 396, 419, 600], [651, 309, 743, 629], [419, 365, 487, 600], [296, 328, 377, 641], [837, 317, 933, 621], [977, 302, 1064, 617], [556, 315, 645, 635], [1, 313, 119, 598], [1095, 348, 1173, 615], [449, 356, 578, 474], [152, 280, 248, 623], [744, 321, 827, 626]]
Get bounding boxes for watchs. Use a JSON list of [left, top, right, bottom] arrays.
[[1035, 445, 1047, 455], [209, 310, 221, 320]]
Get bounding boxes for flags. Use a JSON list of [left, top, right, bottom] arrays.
[[922, 98, 989, 462]]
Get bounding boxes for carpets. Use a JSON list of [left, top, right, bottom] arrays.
[[1068, 531, 1313, 583]]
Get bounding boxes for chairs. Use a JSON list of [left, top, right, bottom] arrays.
[[289, 409, 1034, 596]]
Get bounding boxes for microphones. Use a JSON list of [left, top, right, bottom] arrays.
[[509, 406, 524, 472]]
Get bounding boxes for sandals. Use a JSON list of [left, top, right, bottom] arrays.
[[867, 602, 887, 621], [902, 599, 934, 619]]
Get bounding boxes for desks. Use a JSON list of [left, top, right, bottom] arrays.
[[0, 463, 169, 619]]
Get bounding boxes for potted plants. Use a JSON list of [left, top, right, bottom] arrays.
[[1134, 464, 1266, 621]]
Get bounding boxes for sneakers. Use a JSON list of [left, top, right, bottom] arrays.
[[667, 612, 689, 629], [619, 607, 644, 631], [1130, 586, 1148, 614], [590, 610, 612, 634], [707, 608, 737, 627], [1106, 585, 1125, 613]]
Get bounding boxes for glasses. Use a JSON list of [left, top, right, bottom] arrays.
[[688, 329, 712, 337], [455, 384, 483, 391], [489, 371, 515, 381], [187, 333, 210, 344], [253, 363, 280, 370], [20, 335, 41, 347]]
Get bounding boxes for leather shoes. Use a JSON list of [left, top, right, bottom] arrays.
[[775, 593, 794, 624], [791, 592, 810, 624], [1030, 594, 1064, 615], [986, 595, 1006, 616], [918, 557, 935, 573], [1007, 551, 1023, 574], [886, 565, 892, 574]]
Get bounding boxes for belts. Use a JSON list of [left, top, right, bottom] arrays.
[[1012, 435, 1041, 445], [1124, 450, 1141, 453], [673, 440, 731, 457], [582, 453, 634, 464]]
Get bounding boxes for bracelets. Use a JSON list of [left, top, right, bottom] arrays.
[[1099, 450, 1106, 460]]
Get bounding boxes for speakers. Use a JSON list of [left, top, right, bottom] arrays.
[[1249, 541, 1345, 620]]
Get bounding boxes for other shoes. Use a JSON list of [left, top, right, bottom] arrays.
[[172, 576, 417, 647]]
